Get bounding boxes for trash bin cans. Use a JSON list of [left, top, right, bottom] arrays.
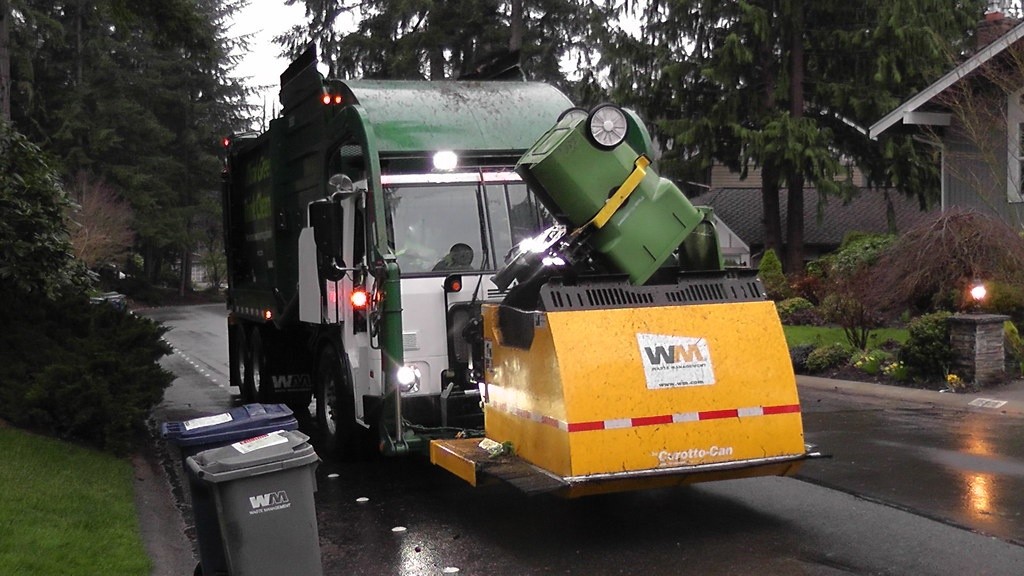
[[160, 404, 323, 576], [513, 104, 727, 289]]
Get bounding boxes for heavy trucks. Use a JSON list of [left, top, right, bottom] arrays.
[[218, 40, 821, 515]]
